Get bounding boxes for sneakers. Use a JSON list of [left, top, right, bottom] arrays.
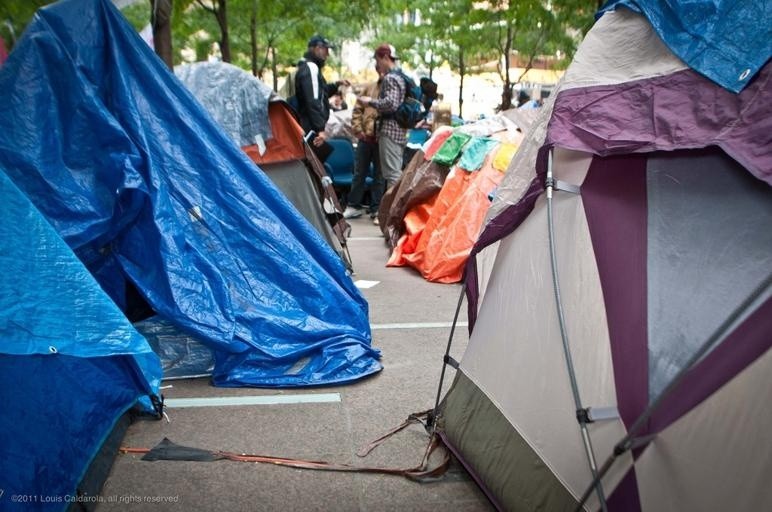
[[342, 205, 380, 225]]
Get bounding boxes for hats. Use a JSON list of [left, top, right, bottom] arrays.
[[308, 35, 335, 50], [374, 42, 402, 60]]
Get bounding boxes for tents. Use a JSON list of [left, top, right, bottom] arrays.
[[379, 108, 542, 284], [428, 0, 772, 512], [174, 60, 354, 275], [0, 0, 384, 512]]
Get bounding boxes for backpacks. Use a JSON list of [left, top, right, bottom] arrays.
[[278, 59, 316, 115], [381, 71, 438, 130]]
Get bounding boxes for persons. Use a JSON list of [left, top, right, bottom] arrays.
[[360, 43, 407, 190], [497, 91, 529, 112], [290, 36, 381, 224]]
